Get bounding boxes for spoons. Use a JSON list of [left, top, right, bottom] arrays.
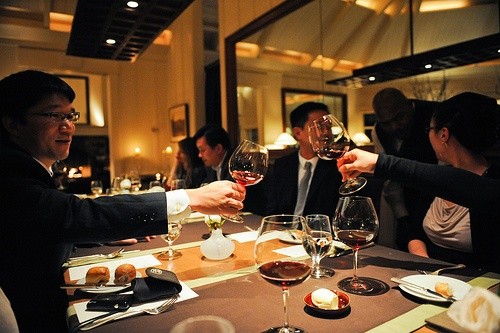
[[69, 247, 125, 265]]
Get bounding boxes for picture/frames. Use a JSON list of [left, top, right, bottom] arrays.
[[55, 75, 91, 126], [168, 103, 189, 142]]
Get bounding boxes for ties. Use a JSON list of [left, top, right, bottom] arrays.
[[292, 162, 312, 229]]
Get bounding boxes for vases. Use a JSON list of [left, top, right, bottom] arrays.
[[201, 228, 235, 260]]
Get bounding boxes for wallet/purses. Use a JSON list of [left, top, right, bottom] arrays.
[[87, 294, 133, 310]]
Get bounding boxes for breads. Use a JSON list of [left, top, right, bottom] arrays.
[[114, 264, 136, 284], [435, 281, 455, 296], [86, 267, 110, 284], [311, 288, 339, 309]]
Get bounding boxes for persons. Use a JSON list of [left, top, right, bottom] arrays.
[[167, 124, 260, 216], [337, 87, 500, 277], [0, 69, 246, 333], [252, 101, 368, 238]]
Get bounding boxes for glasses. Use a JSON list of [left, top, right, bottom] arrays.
[[14, 111, 80, 123], [425, 127, 438, 134]]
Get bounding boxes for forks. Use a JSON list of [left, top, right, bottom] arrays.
[[86, 293, 178, 328]]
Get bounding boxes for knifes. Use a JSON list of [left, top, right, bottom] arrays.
[[59, 285, 127, 290], [390, 276, 457, 302]]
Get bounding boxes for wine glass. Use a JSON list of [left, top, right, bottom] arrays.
[[308, 114, 368, 196], [253, 215, 317, 332], [301, 214, 333, 279], [157, 227, 183, 261], [91, 180, 102, 197], [332, 196, 380, 295], [217, 139, 269, 224]]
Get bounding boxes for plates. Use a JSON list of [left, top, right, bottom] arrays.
[[280, 232, 303, 244], [399, 275, 473, 302], [304, 290, 350, 313], [76, 271, 142, 294]]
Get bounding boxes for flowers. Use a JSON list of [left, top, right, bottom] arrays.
[[204, 213, 225, 232]]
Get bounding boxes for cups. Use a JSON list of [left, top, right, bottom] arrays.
[[111, 177, 123, 194], [170, 179, 187, 191], [149, 181, 161, 190]]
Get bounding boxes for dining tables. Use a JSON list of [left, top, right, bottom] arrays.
[[62, 190, 500, 333]]
